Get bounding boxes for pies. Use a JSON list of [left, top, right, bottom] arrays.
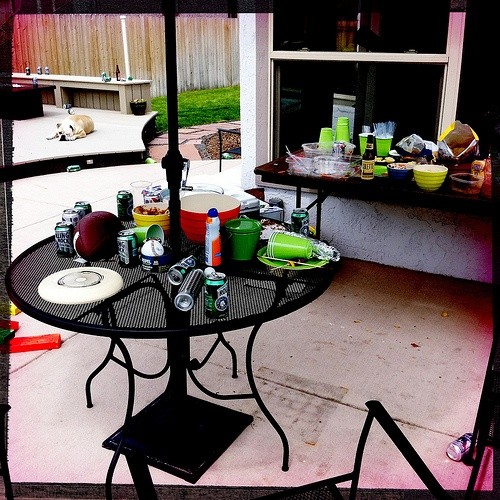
[[135, 206, 169, 215]]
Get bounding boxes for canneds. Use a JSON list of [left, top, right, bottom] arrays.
[[37, 66, 42, 75], [44, 67, 49, 74], [174, 269, 203, 311], [74, 201, 92, 220], [203, 272, 229, 319], [117, 230, 140, 268], [25, 67, 30, 75], [67, 165, 81, 172], [167, 255, 197, 285], [63, 104, 72, 109], [102, 72, 111, 82], [62, 209, 80, 226], [291, 207, 309, 238], [116, 190, 134, 223], [55, 222, 76, 258], [446, 433, 474, 460]]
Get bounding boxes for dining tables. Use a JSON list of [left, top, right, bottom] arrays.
[[253, 147, 497, 230], [4, 205, 339, 500]]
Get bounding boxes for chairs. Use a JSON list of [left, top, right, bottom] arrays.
[[216, 128, 240, 172]]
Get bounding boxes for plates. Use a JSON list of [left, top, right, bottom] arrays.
[[257, 246, 329, 271]]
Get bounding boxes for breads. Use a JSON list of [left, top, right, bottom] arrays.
[[448, 138, 475, 155]]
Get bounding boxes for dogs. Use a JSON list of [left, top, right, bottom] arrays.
[[44, 113, 96, 142]]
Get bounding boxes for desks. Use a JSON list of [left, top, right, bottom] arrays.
[[11, 73, 152, 115], [11, 82, 56, 121]]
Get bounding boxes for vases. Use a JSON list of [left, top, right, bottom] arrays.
[[129, 99, 147, 115]]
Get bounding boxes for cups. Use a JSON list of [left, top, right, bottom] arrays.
[[130, 224, 164, 245], [145, 158, 157, 164], [224, 218, 263, 260], [318, 128, 333, 150], [129, 180, 152, 207], [335, 117, 350, 143], [267, 232, 312, 260]]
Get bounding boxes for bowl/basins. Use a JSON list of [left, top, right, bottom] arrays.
[[131, 203, 170, 236], [374, 165, 388, 177], [387, 163, 414, 180], [413, 165, 449, 191], [160, 183, 224, 203], [180, 193, 241, 245], [359, 133, 394, 156]]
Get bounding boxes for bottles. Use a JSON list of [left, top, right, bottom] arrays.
[[205, 208, 222, 267], [361, 135, 375, 180], [116, 65, 120, 81], [484, 154, 492, 186]]
